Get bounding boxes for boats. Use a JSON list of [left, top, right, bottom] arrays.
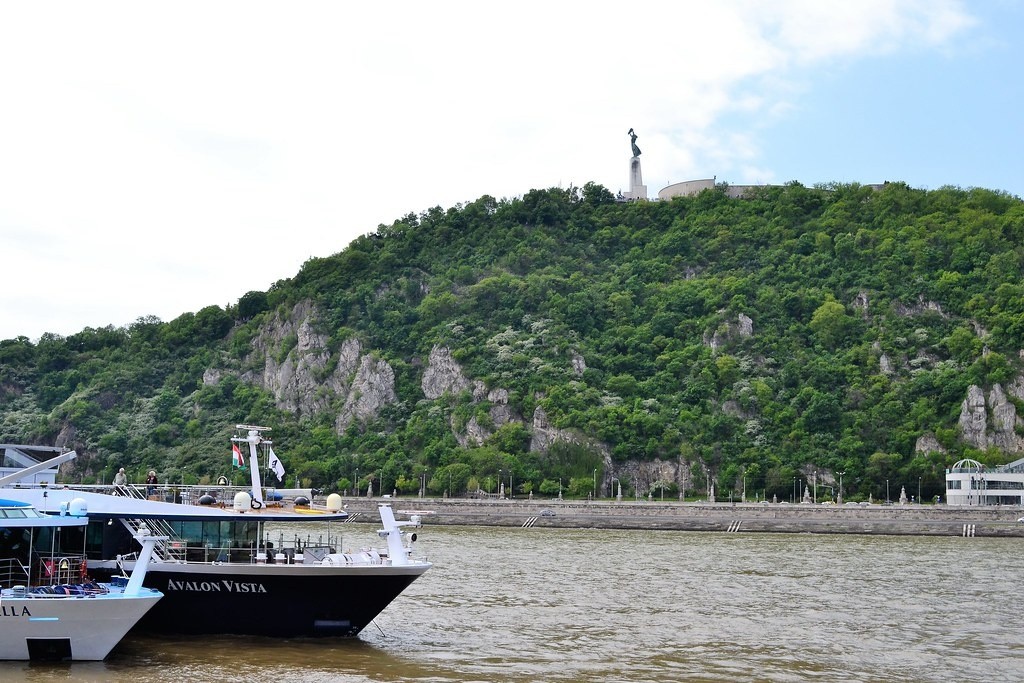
[[0, 424, 434, 637], [0, 498, 170, 663]]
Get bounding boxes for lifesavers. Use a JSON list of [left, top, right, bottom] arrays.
[[80, 560, 88, 578]]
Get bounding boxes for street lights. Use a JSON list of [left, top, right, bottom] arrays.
[[498, 469, 501, 494], [510, 471, 512, 498], [594, 469, 597, 498], [814, 471, 816, 503], [918, 476, 921, 504], [794, 477, 796, 503], [798, 479, 801, 503], [744, 472, 747, 503], [707, 469, 709, 501], [836, 472, 845, 504], [887, 480, 889, 504]]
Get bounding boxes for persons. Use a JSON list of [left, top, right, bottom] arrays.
[[146, 471, 158, 500], [113, 468, 127, 496]]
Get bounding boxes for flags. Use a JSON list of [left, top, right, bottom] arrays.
[[269, 447, 285, 482], [233, 444, 245, 469]]
